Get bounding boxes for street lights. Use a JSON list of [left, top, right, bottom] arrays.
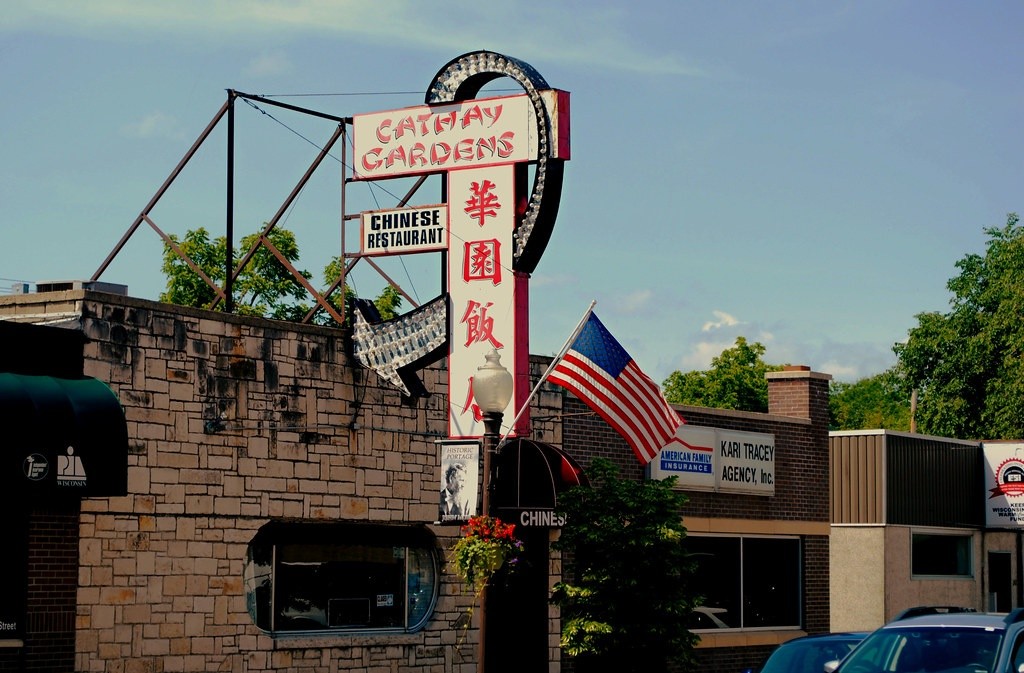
[[472, 345, 513, 673]]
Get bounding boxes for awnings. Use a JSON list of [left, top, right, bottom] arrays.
[[1, 365, 128, 498]]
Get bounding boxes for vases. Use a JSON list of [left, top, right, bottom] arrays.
[[487, 548, 504, 569]]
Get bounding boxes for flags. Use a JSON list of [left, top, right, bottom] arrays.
[[546, 312, 687, 465]]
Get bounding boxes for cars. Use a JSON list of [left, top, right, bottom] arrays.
[[747, 630, 916, 673], [821, 604, 1024, 673]]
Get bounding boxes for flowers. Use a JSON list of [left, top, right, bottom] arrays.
[[448, 514, 524, 597]]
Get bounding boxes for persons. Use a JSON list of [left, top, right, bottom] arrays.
[[439, 463, 470, 517]]
